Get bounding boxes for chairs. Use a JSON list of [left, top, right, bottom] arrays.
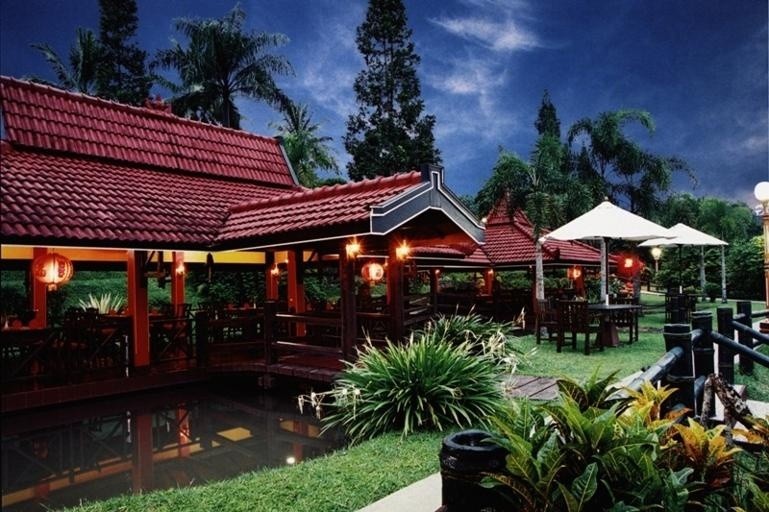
[[0, 288, 641, 386]]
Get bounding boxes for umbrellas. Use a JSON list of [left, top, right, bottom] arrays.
[[537, 196, 680, 294], [637, 223, 729, 286]]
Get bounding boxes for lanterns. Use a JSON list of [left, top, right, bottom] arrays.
[[565, 268, 581, 280], [360, 263, 385, 288], [30, 252, 74, 291]]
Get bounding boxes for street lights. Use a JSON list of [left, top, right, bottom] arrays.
[[754, 181, 769, 309], [651, 247, 662, 283]]
